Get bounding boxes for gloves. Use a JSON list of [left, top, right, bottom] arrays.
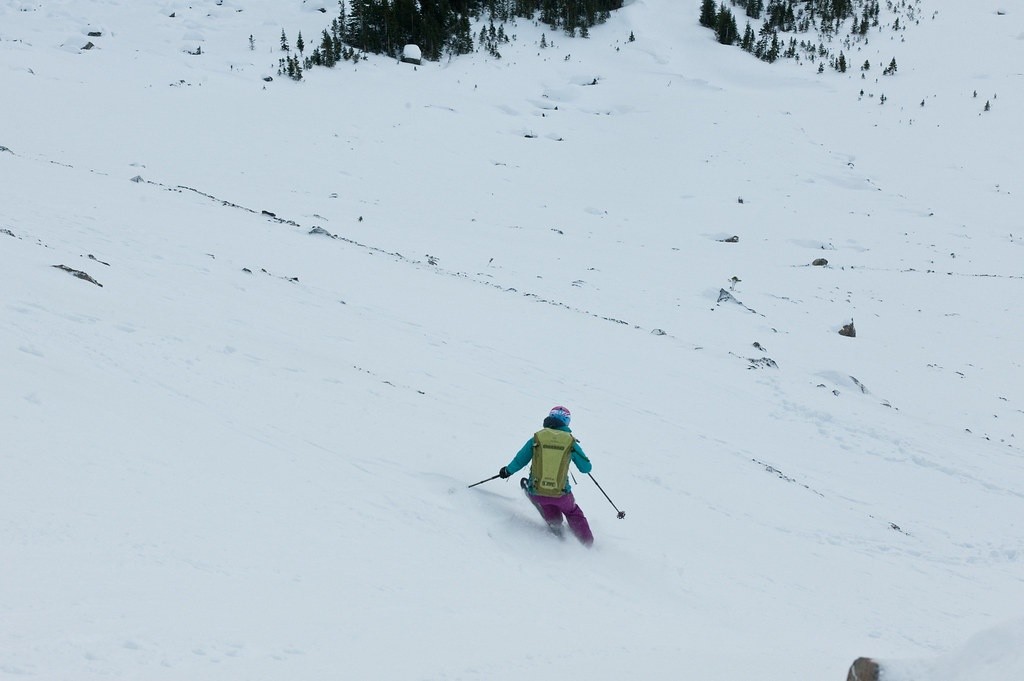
[[499, 467, 511, 479]]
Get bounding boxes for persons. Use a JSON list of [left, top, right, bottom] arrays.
[[499, 405, 595, 548], [847, 658, 879, 681]]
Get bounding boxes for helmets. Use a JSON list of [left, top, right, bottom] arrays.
[[549, 406, 571, 426]]
